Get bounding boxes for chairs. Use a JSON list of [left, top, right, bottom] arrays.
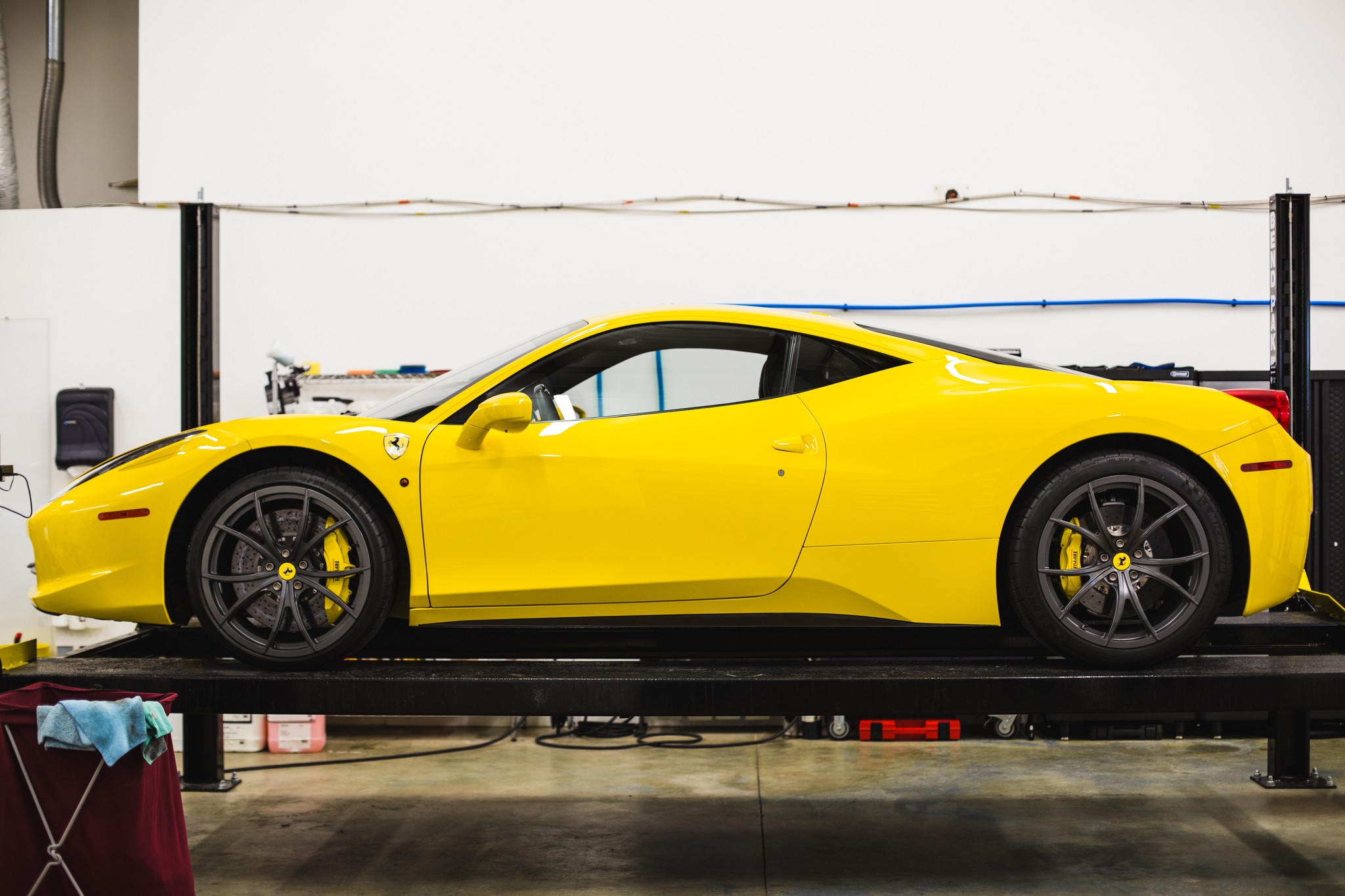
[[759, 338, 786, 398]]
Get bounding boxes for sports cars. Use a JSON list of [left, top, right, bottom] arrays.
[[23, 303, 1312, 671]]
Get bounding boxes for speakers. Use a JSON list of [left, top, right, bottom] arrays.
[[56, 387, 115, 470]]
